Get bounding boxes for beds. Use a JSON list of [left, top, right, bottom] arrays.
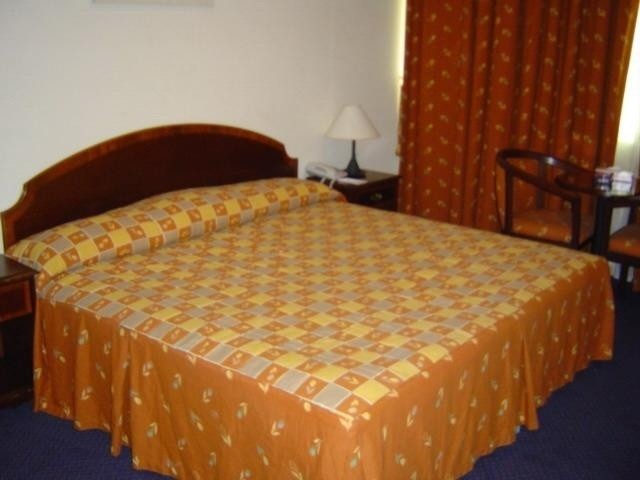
[[0, 123, 617, 480]]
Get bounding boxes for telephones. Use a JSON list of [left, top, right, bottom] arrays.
[[306, 161, 349, 180]]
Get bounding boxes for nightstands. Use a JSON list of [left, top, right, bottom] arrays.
[[0, 254, 39, 411], [306, 170, 401, 211]]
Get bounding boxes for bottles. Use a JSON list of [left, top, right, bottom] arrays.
[[593, 169, 612, 192]]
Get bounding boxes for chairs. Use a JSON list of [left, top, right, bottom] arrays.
[[602, 194, 640, 292], [495, 148, 594, 249]]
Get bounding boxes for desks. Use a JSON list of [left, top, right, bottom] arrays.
[[554, 172, 605, 255]]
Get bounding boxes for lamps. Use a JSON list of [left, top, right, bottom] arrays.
[[326, 105, 380, 178]]
